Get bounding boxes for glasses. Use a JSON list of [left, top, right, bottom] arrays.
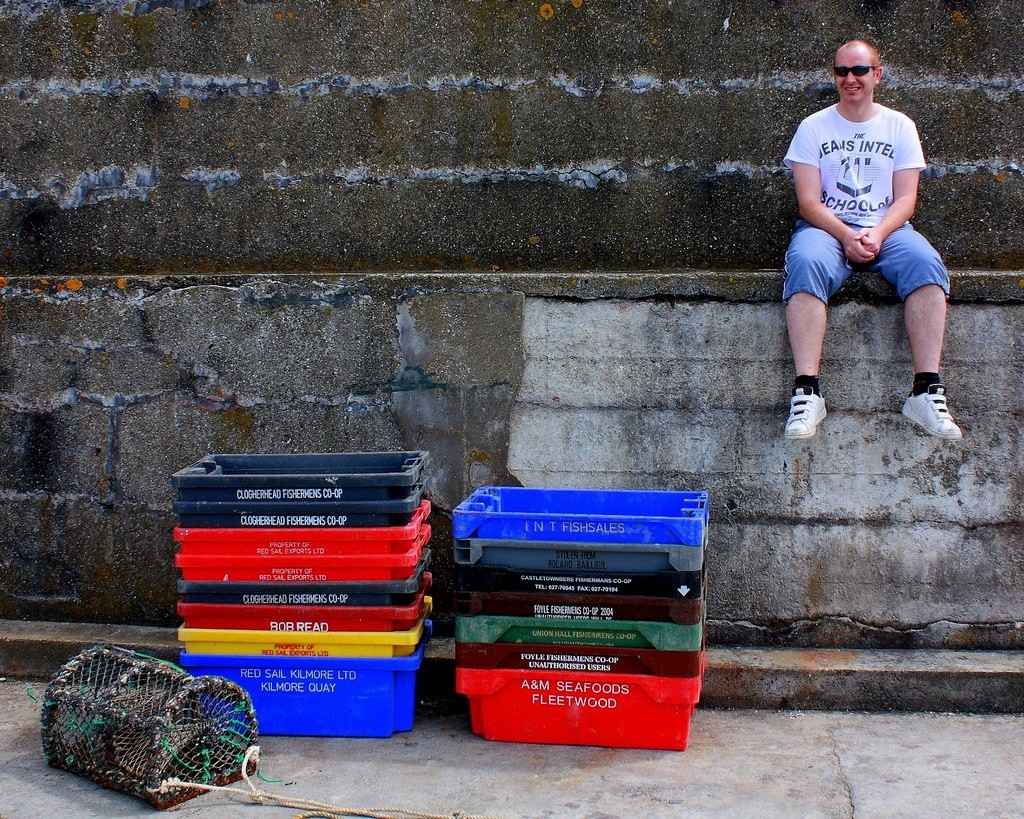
[[832, 65, 878, 77]]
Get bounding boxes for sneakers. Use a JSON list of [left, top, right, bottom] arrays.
[[784, 386, 827, 439], [901, 383, 962, 439]]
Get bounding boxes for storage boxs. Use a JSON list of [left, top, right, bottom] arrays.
[[449, 484, 712, 753], [166, 450, 435, 738]]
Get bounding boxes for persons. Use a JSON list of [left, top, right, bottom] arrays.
[[783, 40, 963, 440]]
